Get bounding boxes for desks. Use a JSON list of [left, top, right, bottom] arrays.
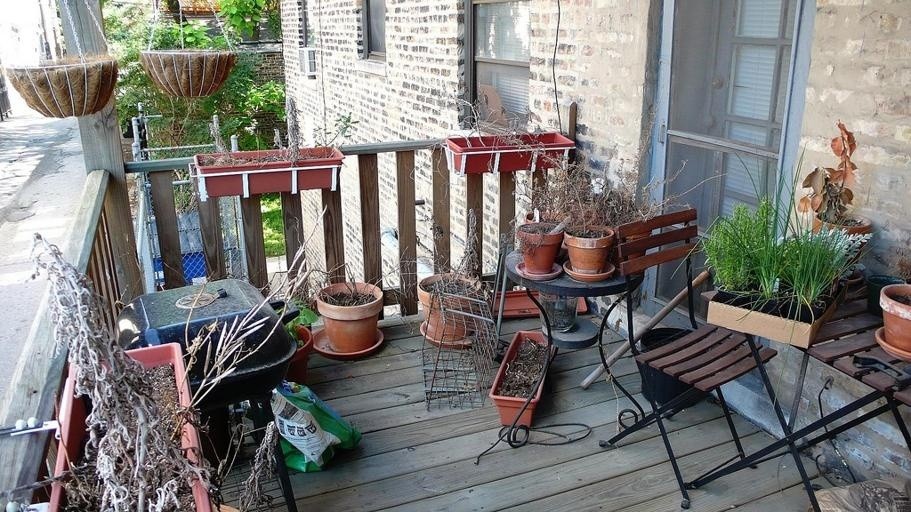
[[505, 250, 644, 448], [684, 289, 911, 511]]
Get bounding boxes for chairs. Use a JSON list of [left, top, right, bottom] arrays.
[[600, 207, 780, 511]]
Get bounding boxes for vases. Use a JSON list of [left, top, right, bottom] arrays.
[[446, 131, 577, 176], [5, 55, 117, 120], [490, 330, 552, 431], [869, 275, 911, 364]]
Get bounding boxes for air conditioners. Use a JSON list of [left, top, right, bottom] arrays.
[[298, 47, 317, 77]]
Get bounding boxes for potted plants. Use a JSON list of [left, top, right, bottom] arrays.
[[141, 0, 270, 99], [189, 99, 361, 203], [701, 119, 875, 349], [261, 247, 416, 386], [417, 204, 484, 350], [514, 139, 730, 282], [33, 207, 331, 512]]
